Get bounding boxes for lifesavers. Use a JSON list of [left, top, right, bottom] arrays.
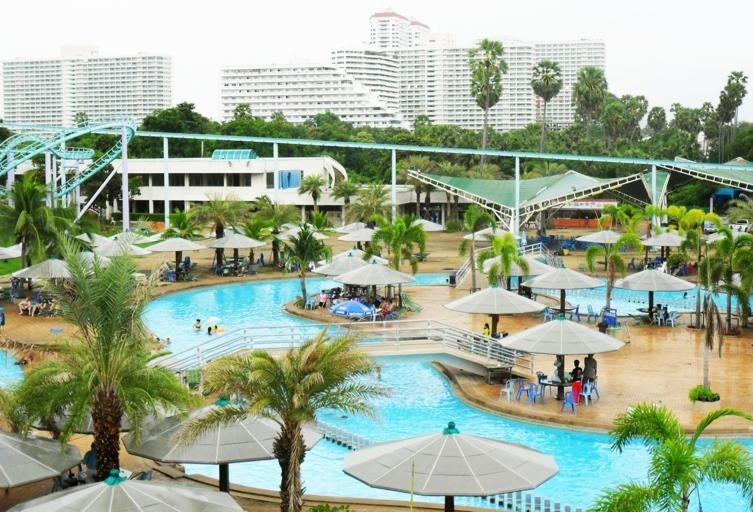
[[211, 324, 224, 336]]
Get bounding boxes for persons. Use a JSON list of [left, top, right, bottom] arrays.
[[193, 318, 218, 335], [571, 353, 598, 399], [11, 277, 59, 317], [319, 288, 393, 321], [482, 323, 489, 343]]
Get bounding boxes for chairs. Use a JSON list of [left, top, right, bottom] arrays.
[[628, 257, 687, 276], [544, 303, 677, 328], [499, 368, 600, 417], [50, 464, 153, 492], [136, 253, 265, 287], [305, 289, 424, 326]]
